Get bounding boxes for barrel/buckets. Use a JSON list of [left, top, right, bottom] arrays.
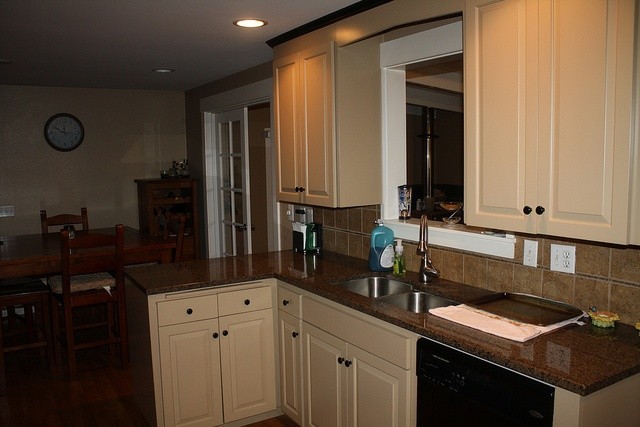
[[368, 221, 395, 272]]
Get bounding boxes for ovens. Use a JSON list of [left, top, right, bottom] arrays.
[[416, 337, 555, 426]]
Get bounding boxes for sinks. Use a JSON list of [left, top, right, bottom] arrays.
[[339, 276, 415, 297], [383, 291, 461, 314]]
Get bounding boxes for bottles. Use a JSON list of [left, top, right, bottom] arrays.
[[160, 160, 189, 178], [305, 223, 324, 253], [398, 185, 412, 219], [64, 225, 76, 251], [305, 253, 323, 278]]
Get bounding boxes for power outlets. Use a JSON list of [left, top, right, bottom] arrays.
[[550, 244, 576, 275], [0, 205, 16, 218], [307, 208, 313, 223], [299, 206, 306, 223], [522, 239, 539, 268], [287, 204, 295, 222]]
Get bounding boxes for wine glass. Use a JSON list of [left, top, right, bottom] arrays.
[[440, 200, 463, 229]]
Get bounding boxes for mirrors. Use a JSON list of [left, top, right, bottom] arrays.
[[405, 51, 464, 222]]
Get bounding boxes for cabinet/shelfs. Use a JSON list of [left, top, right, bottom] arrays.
[[276, 279, 303, 427], [122, 278, 281, 427], [303, 288, 417, 427], [462, 0, 640, 248], [273, 39, 383, 210], [133, 177, 199, 264]]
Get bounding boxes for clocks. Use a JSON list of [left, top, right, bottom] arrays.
[[44, 113, 85, 152]]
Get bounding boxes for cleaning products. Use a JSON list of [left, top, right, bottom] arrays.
[[393, 238, 406, 276]]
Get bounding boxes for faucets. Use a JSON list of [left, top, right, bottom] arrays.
[[416, 214, 441, 283]]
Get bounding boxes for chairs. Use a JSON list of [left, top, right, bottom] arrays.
[[0, 278, 58, 385], [40, 207, 89, 233], [161, 211, 188, 263], [47, 224, 130, 379]]
